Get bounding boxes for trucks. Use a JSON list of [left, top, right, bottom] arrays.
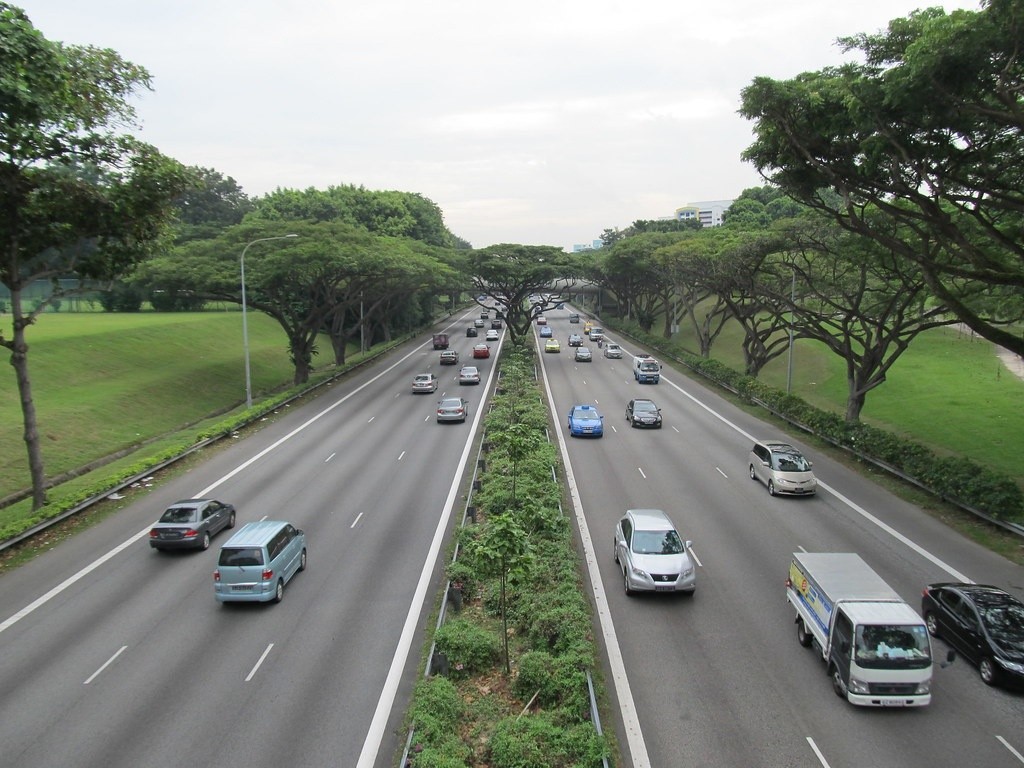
[[787, 553, 957, 708], [432, 333, 449, 350]]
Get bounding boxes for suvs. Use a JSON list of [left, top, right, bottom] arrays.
[[613, 508, 697, 597]]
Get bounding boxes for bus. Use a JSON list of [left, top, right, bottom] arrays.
[[633, 354, 663, 385]]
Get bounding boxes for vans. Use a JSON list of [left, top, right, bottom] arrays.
[[213, 522, 307, 604], [589, 326, 605, 341]]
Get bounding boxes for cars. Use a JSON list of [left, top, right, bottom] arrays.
[[545, 338, 560, 353], [473, 345, 491, 358], [466, 327, 478, 337], [575, 347, 593, 362], [486, 330, 499, 340], [584, 323, 594, 335], [475, 295, 508, 329], [748, 440, 817, 497], [440, 350, 460, 364], [412, 374, 438, 393], [568, 405, 605, 438], [538, 326, 552, 337], [569, 333, 583, 347], [528, 293, 565, 325], [604, 343, 622, 358], [436, 397, 469, 423], [149, 498, 236, 552], [459, 366, 481, 384], [920, 584, 1024, 686], [570, 313, 580, 323], [626, 399, 663, 428]]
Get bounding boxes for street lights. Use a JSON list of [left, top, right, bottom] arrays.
[[239, 233, 299, 411]]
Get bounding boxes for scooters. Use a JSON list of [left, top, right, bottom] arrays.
[[597, 339, 603, 348]]
